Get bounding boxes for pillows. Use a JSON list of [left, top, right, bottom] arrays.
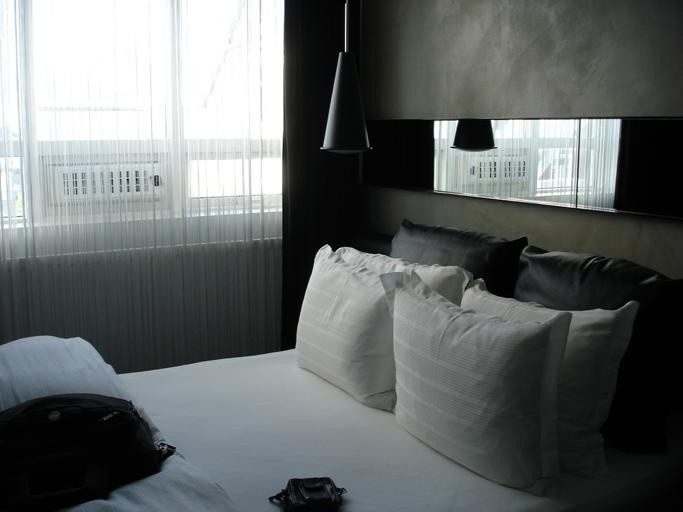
[[296, 241, 409, 417], [375, 266, 576, 493], [514, 242, 681, 462], [454, 274, 642, 489], [335, 243, 474, 313], [392, 223, 529, 301]]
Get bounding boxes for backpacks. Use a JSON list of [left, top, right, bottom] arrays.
[[0, 393, 177, 512]]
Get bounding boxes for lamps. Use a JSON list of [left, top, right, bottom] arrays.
[[320, 0, 373, 154], [451, 120, 497, 151]]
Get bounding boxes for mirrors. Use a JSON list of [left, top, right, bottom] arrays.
[[353, 117, 683, 220]]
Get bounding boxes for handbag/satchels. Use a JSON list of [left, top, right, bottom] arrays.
[[268, 477, 347, 512]]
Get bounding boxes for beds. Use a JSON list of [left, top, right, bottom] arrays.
[[0, 232, 683, 511]]
